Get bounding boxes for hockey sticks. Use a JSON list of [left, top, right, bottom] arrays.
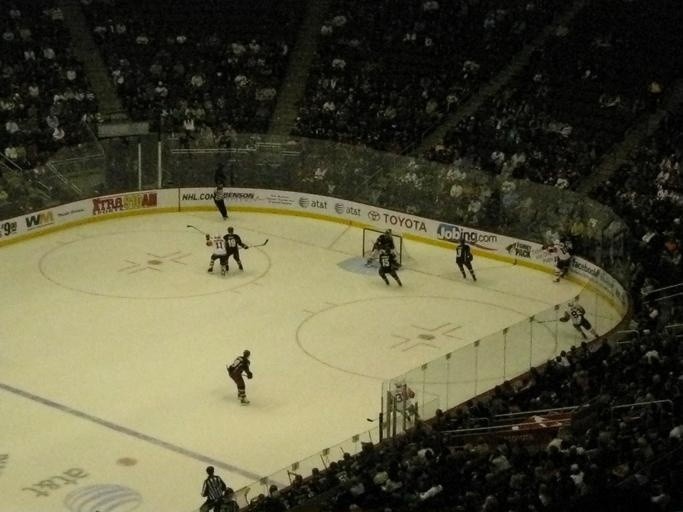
[[366, 412, 394, 423], [244, 487, 251, 505], [288, 472, 299, 483], [187, 225, 212, 239], [225, 364, 248, 376], [238, 239, 268, 248]]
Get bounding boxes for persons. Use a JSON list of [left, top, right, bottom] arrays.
[[284, 3, 682, 288], [205, 227, 248, 277], [202, 284, 683, 512], [1, 2, 298, 219]]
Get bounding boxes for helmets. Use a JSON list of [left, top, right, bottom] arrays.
[[214, 228, 234, 238], [205, 466, 213, 478], [241, 351, 249, 358]]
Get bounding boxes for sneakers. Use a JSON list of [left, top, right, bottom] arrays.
[[207, 264, 241, 275]]
